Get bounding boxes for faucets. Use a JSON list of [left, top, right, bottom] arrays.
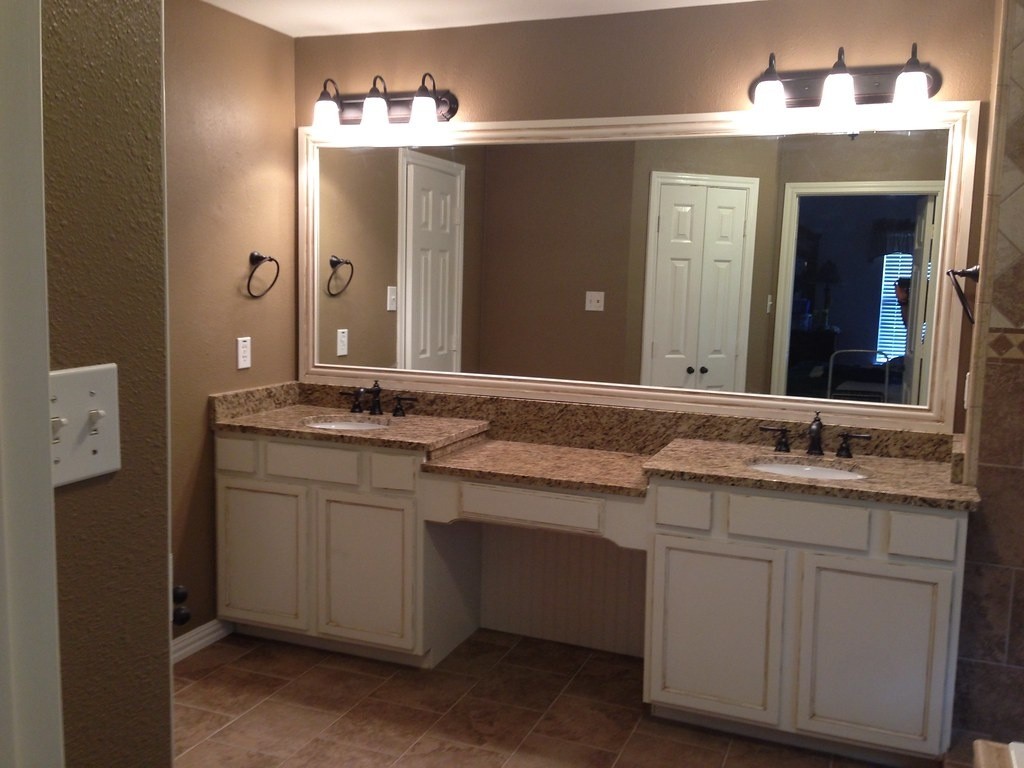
[[809, 410, 824, 455], [365, 380, 384, 414]]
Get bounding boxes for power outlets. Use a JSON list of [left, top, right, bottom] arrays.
[[237, 337, 251, 369], [337, 330, 348, 356], [585, 291, 604, 312]]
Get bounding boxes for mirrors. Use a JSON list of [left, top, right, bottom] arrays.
[[299, 101, 984, 436], [328, 255, 354, 297], [247, 251, 279, 298]]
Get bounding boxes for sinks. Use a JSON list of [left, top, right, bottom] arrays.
[[742, 455, 874, 481], [301, 415, 394, 430]]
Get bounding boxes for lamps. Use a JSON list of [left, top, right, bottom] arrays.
[[752, 52, 788, 118], [819, 47, 859, 116], [358, 75, 392, 130], [310, 78, 343, 136], [410, 72, 441, 130], [892, 41, 929, 114]]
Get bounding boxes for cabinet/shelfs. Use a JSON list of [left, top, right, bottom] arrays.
[[643, 476, 967, 768], [213, 431, 482, 668]]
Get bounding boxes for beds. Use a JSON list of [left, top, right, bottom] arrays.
[[826, 348, 890, 401]]
